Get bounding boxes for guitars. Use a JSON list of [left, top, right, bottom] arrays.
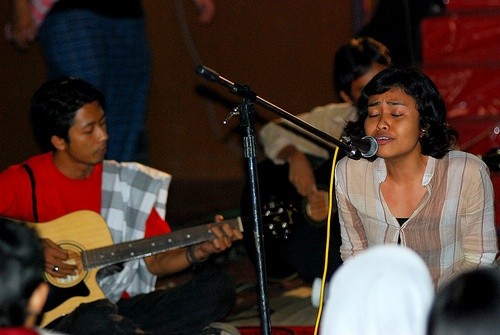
[[11, 202, 299, 331]]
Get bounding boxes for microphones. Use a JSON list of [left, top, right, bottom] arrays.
[[342, 136, 378, 158]]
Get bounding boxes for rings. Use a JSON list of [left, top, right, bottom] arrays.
[[53, 265, 59, 272]]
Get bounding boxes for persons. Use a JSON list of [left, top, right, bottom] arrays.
[[0, 77, 246, 335], [258, 37, 392, 310], [334, 64, 498, 296], [0, 215, 50, 335], [11, 0, 217, 161]]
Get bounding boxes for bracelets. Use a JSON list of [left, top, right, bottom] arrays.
[[190, 245, 208, 264], [186, 247, 195, 266]]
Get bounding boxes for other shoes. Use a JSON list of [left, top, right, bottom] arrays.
[[199, 322, 241, 335]]
